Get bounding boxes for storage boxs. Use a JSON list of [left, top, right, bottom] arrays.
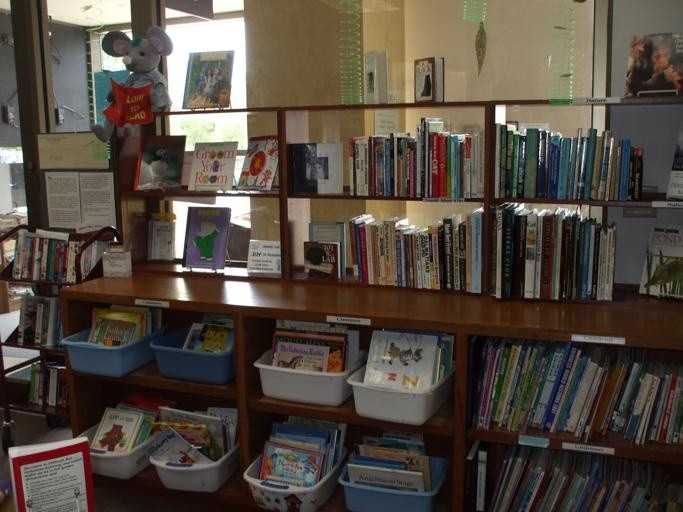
[[253, 347, 366, 406], [60, 322, 163, 377], [77, 420, 159, 480], [242, 445, 345, 512], [149, 327, 235, 384], [148, 436, 237, 493], [346, 359, 455, 426], [337, 455, 447, 511]]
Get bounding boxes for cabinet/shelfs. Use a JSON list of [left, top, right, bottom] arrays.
[[108, 83, 682, 307], [56, 276, 682, 512], [1, 223, 122, 429]]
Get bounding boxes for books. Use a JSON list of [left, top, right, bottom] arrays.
[[182, 47, 235, 112], [287, 117, 486, 294], [90, 304, 237, 466], [9, 437, 95, 512], [489, 124, 682, 302], [133, 134, 278, 270], [465, 339, 683, 512], [363, 49, 390, 104], [627, 32, 682, 98], [259, 318, 457, 493], [413, 54, 447, 104], [12, 230, 112, 407]]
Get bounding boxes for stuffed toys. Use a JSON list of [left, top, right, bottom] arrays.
[[87, 27, 175, 144]]
